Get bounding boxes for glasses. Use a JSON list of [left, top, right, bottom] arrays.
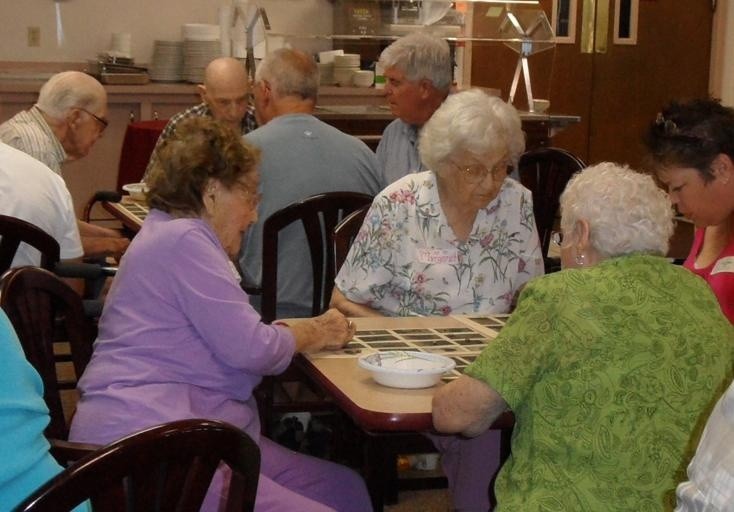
[[655, 111, 715, 142], [70, 105, 111, 133], [549, 228, 576, 250], [451, 159, 508, 182]]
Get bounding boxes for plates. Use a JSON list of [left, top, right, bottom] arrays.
[[149, 22, 374, 87]]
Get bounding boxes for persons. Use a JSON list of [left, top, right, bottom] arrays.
[[68, 113, 374, 512], [0, 139, 87, 296], [328, 88, 550, 510], [0, 304, 98, 512], [220, 48, 373, 330], [672, 376, 734, 511], [639, 93, 734, 321], [373, 30, 454, 195], [430, 160, 734, 512], [0, 72, 132, 257], [131, 54, 260, 184]]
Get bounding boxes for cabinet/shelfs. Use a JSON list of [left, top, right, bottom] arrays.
[[59, 94, 207, 221]]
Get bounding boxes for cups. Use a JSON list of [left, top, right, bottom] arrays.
[[353, 15, 375, 35], [534, 99, 550, 114], [110, 32, 131, 53]]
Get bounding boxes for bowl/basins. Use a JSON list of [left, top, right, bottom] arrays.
[[390, 25, 461, 38], [121, 183, 149, 202], [359, 351, 456, 390]]
